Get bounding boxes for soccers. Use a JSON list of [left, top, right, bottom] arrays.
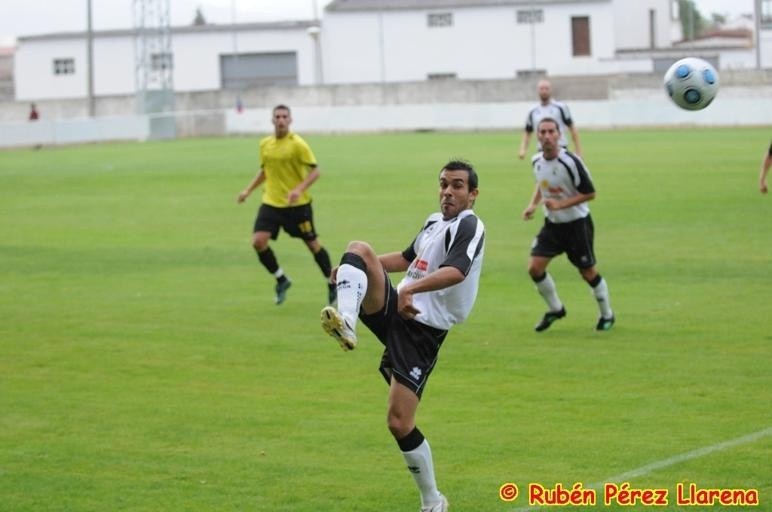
[[664, 57, 718, 110]]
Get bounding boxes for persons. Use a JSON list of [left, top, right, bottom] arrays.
[[521, 118, 615, 336], [517, 79, 581, 159], [758, 143, 771, 193], [321, 160, 485, 512], [29, 103, 43, 150], [235, 103, 338, 306]]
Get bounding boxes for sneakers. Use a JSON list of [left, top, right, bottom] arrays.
[[276, 281, 291, 304], [328, 282, 337, 304], [320, 306, 356, 353], [597, 316, 614, 330], [535, 306, 566, 332]]
